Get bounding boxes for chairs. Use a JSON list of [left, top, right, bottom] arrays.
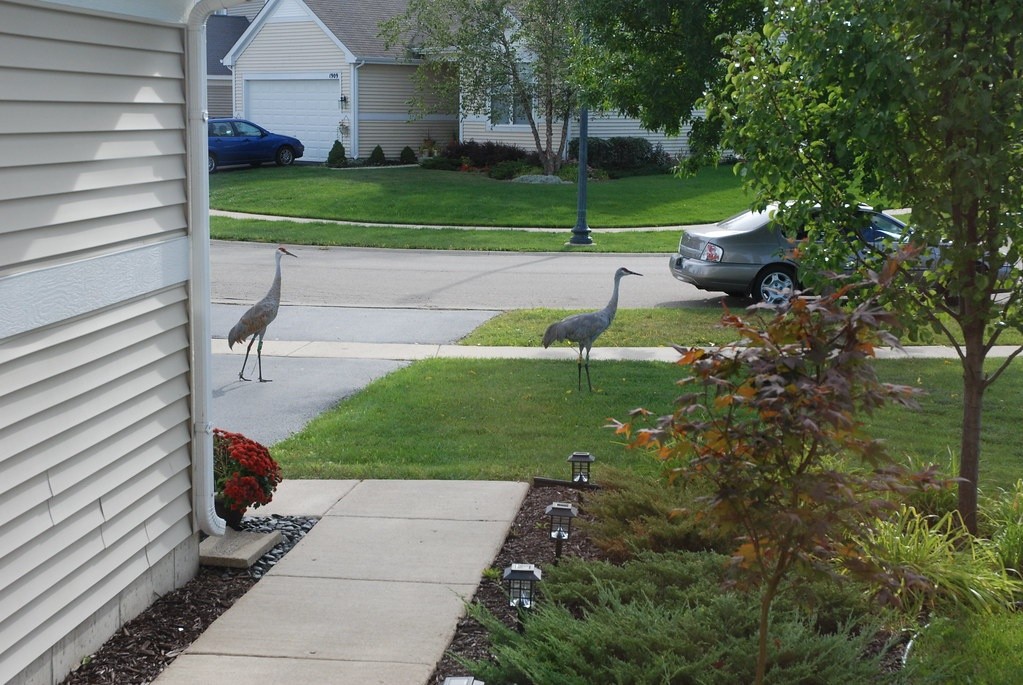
[[219, 126, 228, 137], [208, 125, 220, 137]]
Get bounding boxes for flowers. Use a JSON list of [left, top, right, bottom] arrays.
[[213, 426, 282, 517]]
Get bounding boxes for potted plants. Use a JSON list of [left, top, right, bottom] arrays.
[[419, 144, 430, 157], [433, 145, 438, 157], [423, 138, 433, 147]]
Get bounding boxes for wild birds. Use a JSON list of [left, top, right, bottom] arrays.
[[541, 267, 644, 393], [229, 247, 299, 381]]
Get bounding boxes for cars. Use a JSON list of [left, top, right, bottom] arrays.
[[207, 117, 304, 174], [668, 200, 1018, 314]]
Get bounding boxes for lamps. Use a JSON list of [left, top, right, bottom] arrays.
[[499, 563, 543, 632], [568, 450, 594, 493], [544, 503, 579, 562]]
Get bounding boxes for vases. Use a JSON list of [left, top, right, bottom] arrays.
[[215, 499, 244, 529]]
